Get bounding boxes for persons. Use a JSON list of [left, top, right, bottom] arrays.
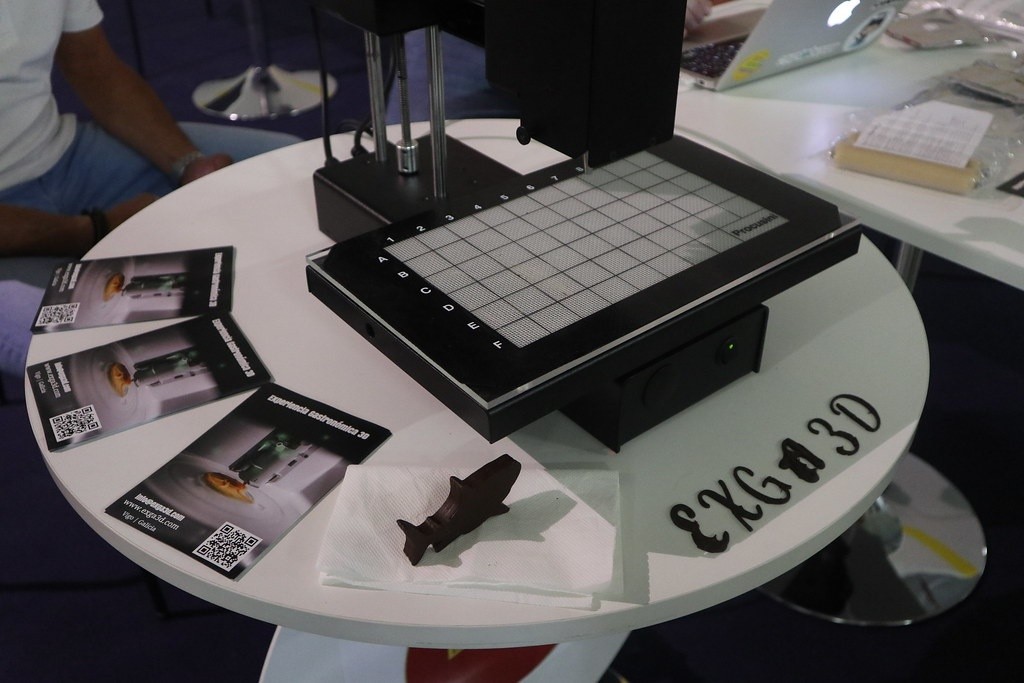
[[683, 0, 711, 40], [0, 0, 304, 298]]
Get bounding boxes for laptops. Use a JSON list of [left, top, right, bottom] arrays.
[[679, 0, 910, 92]]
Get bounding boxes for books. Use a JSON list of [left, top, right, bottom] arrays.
[[885, 7, 996, 48], [948, 51, 1024, 105]]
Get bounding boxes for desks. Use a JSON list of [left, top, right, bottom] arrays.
[[672, 1, 1023, 295], [24, 119, 929, 683]]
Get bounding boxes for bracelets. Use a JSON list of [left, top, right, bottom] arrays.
[[170, 150, 205, 180], [83, 207, 111, 242]]
